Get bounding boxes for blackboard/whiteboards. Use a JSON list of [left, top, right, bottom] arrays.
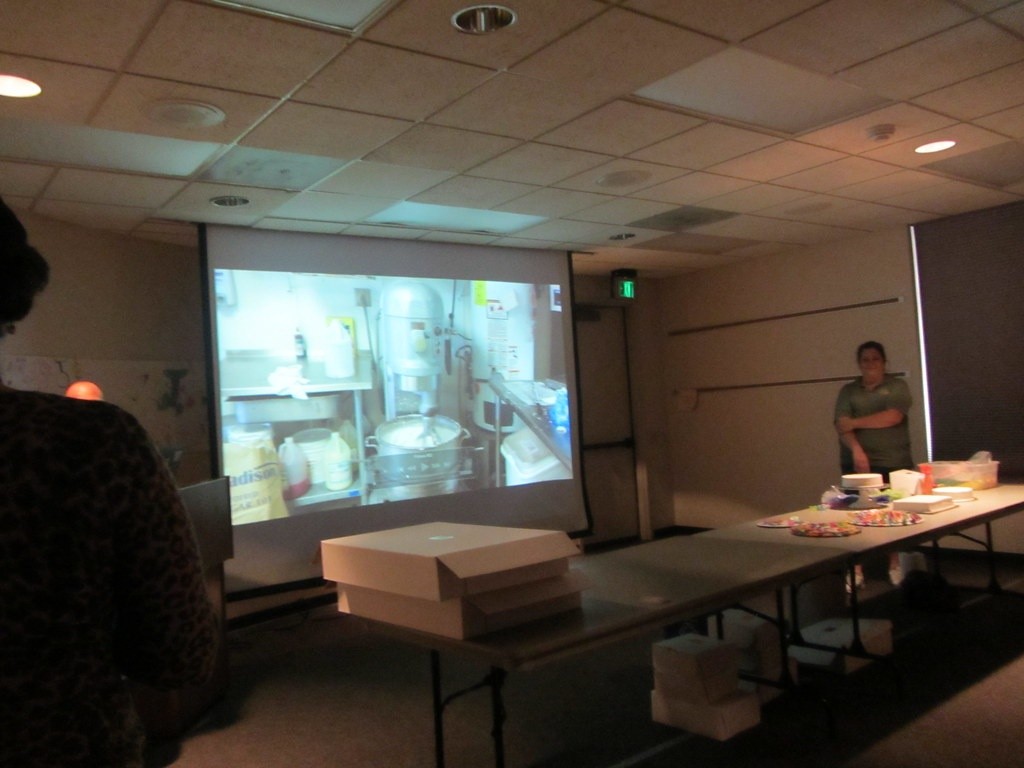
[[909, 198, 1024, 484]]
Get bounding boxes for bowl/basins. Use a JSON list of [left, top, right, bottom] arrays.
[[933, 487, 973, 498]]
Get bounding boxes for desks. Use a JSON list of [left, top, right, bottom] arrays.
[[692, 485, 1024, 715], [382, 538, 849, 768]]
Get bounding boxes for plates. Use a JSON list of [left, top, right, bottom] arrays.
[[756, 521, 787, 527], [789, 514, 923, 536], [952, 496, 976, 503]]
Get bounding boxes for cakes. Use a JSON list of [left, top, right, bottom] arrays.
[[841, 474, 884, 488], [892, 495, 954, 513], [931, 487, 974, 500]]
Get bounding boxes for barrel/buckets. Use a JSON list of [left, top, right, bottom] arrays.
[[230, 422, 332, 483]]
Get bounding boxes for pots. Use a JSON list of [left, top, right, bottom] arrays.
[[365, 414, 472, 496]]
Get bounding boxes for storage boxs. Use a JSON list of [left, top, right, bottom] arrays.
[[781, 617, 894, 674], [652, 632, 734, 677], [650, 690, 760, 742], [928, 459, 1001, 490], [336, 567, 581, 639], [655, 670, 737, 706], [320, 519, 582, 606]]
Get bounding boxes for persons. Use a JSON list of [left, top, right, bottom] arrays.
[[834, 341, 914, 592], [0, 197, 217, 767]]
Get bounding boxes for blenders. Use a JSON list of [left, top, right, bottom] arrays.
[[367, 281, 458, 505]]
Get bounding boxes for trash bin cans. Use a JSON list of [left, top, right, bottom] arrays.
[[500, 428, 569, 487]]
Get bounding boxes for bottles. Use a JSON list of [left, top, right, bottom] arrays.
[[919, 465, 933, 494], [324, 319, 354, 377], [321, 432, 352, 490], [277, 437, 310, 501], [295, 329, 305, 359], [338, 419, 360, 472]]
